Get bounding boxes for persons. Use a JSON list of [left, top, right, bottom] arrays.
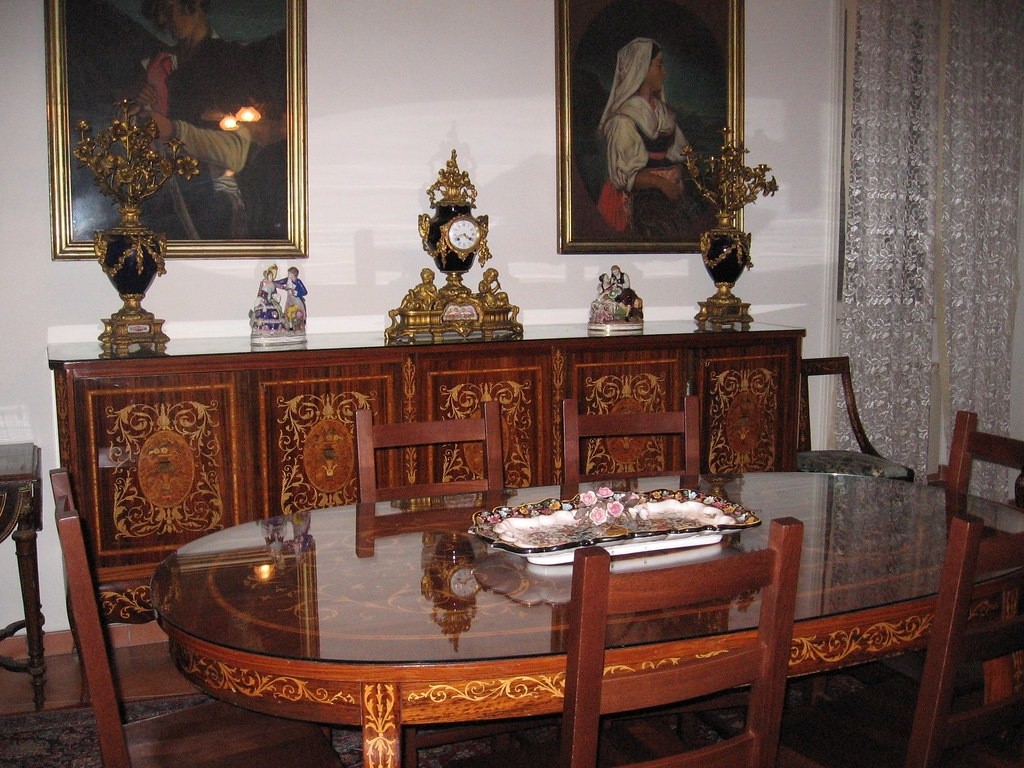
[[252, 263, 308, 332], [397, 267, 437, 311], [588, 265, 645, 325], [478, 267, 516, 309]]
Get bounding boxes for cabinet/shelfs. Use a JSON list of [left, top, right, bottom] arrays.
[[46, 320, 806, 704]]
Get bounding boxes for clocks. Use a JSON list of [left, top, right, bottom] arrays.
[[445, 215, 482, 254]]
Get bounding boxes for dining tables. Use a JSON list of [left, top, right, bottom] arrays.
[[151, 472, 1023, 768]]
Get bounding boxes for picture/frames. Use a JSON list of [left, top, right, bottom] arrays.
[[554, 0, 745, 253], [43, 0, 308, 262]]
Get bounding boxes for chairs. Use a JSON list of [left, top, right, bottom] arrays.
[[356, 489, 505, 558], [796, 356, 914, 482], [780, 512, 1024, 768], [356, 401, 505, 502], [50, 467, 344, 768], [442, 517, 804, 768], [946, 411, 1024, 510], [563, 396, 701, 481]]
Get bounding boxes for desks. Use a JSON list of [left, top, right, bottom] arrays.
[[0, 443, 46, 712]]
